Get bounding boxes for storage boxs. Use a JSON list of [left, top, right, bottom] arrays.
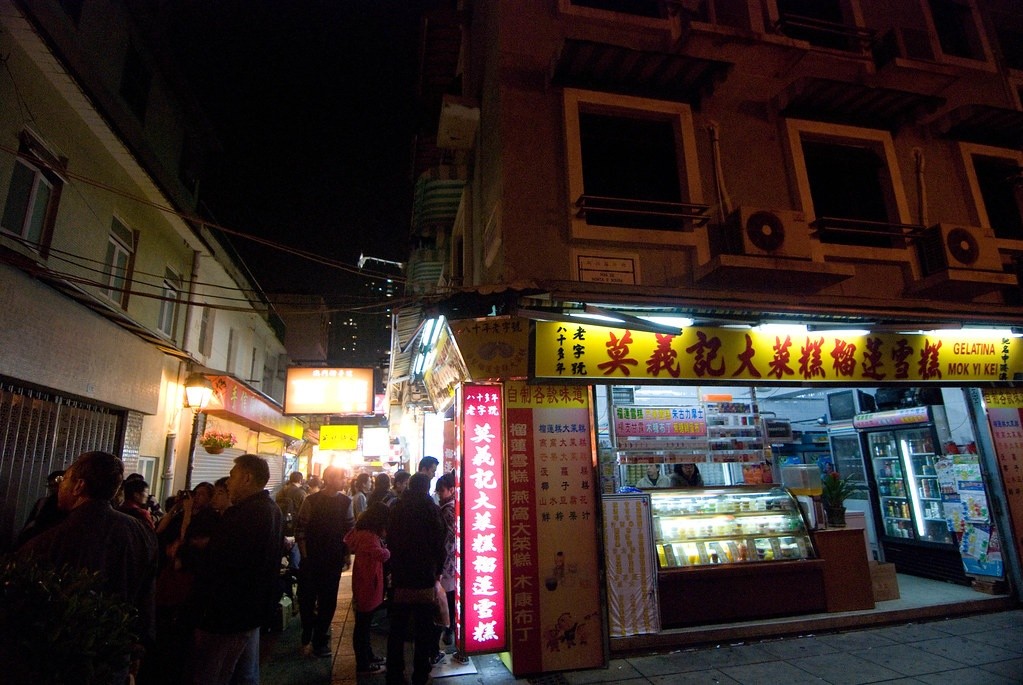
[[868, 559, 900, 603]]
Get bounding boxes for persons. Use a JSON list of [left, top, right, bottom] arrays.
[[669, 463, 704, 489], [635, 464, 668, 487], [0, 454, 458, 685]]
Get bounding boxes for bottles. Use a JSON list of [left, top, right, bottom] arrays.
[[924, 501, 943, 518], [877, 460, 902, 478], [834, 440, 860, 459], [843, 484, 868, 500], [717, 403, 750, 413], [871, 434, 898, 456], [879, 480, 906, 497], [886, 500, 911, 518], [912, 456, 941, 475], [887, 520, 913, 538], [900, 430, 935, 454], [927, 524, 951, 542], [917, 479, 942, 498], [838, 461, 865, 482]]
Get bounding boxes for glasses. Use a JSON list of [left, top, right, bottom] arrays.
[[54, 475, 79, 483]]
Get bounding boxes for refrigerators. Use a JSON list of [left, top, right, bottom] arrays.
[[853, 405, 970, 587], [828, 428, 884, 564]]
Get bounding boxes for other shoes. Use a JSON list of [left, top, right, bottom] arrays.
[[443, 631, 454, 644], [311, 646, 332, 657], [302, 627, 312, 644]]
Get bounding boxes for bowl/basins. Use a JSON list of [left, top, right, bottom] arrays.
[[693, 500, 724, 513], [676, 554, 702, 565], [756, 539, 774, 560], [737, 517, 797, 533], [659, 501, 692, 513], [725, 499, 751, 513], [669, 525, 732, 538], [778, 537, 800, 558], [726, 546, 749, 562]]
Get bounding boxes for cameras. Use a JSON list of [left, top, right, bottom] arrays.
[[178, 490, 189, 500]]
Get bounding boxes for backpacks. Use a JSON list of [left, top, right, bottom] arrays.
[[278, 487, 302, 516]]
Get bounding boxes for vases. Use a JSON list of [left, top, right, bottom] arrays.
[[824, 506, 846, 527]]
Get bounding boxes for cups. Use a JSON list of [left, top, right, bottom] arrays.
[[711, 554, 718, 563]]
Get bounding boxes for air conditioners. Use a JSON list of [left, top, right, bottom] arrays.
[[729, 206, 814, 262], [882, 24, 943, 67], [920, 223, 1003, 280]]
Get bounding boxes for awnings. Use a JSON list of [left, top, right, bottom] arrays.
[[413, 164, 468, 230], [406, 249, 445, 303], [388, 306, 424, 385]]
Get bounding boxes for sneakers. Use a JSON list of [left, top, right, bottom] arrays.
[[429, 649, 447, 666], [370, 654, 387, 664], [357, 664, 385, 674], [452, 651, 470, 664]]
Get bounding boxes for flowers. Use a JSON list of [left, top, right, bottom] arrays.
[[818, 469, 872, 507], [200, 430, 239, 448]]
